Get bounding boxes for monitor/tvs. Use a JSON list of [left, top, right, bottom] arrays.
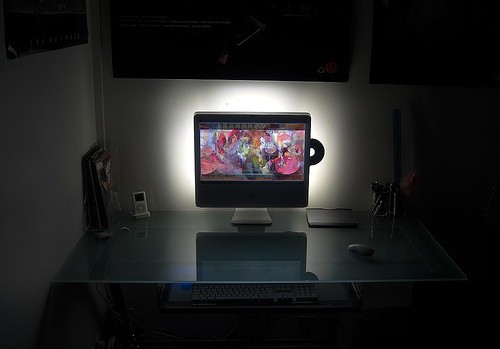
[[195, 114, 311, 225], [195, 224, 308, 280]]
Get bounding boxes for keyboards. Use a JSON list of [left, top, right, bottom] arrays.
[[190, 283, 321, 306]]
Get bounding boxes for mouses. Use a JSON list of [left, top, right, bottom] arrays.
[[348, 244, 375, 256]]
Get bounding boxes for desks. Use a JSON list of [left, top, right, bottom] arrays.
[[52, 210, 467, 349]]
